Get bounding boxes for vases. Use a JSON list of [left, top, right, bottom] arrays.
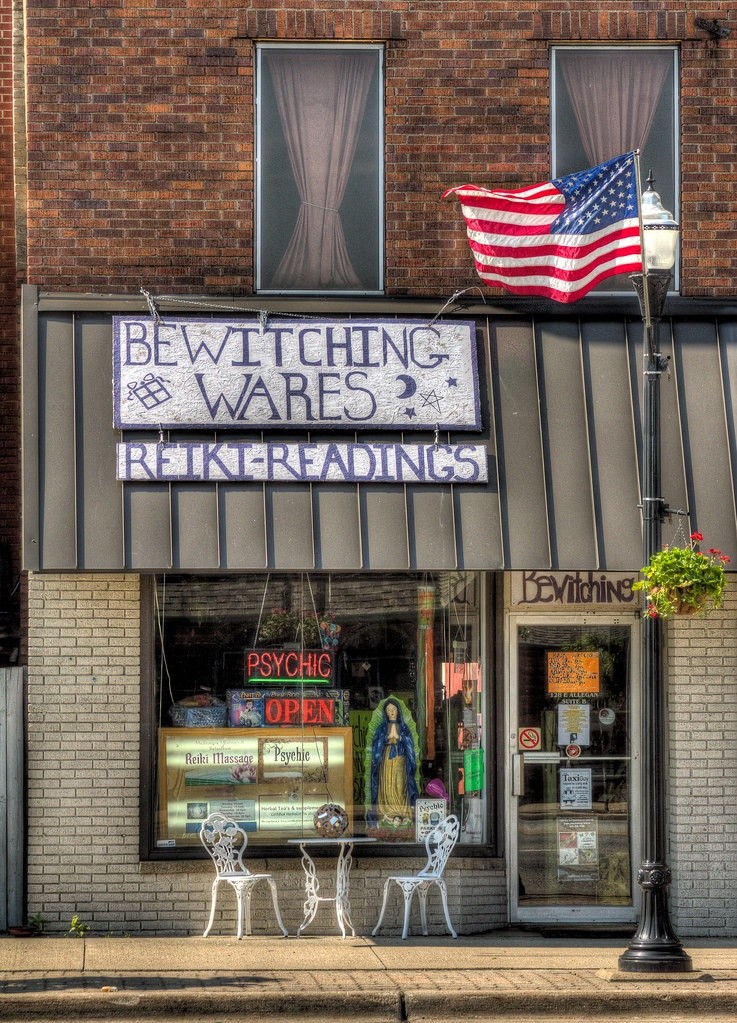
[[649, 587, 706, 615]]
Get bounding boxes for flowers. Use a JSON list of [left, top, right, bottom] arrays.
[[630, 530, 731, 620]]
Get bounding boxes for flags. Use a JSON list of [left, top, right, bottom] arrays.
[[440, 153, 645, 303]]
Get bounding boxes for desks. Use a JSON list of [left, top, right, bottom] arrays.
[[287, 837, 378, 937]]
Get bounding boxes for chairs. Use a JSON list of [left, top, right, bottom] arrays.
[[371, 814, 460, 940], [199, 812, 288, 940]]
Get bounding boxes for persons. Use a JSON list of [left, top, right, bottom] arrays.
[[367, 699, 420, 826]]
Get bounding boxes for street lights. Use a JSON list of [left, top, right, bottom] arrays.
[[620, 167, 694, 971]]
